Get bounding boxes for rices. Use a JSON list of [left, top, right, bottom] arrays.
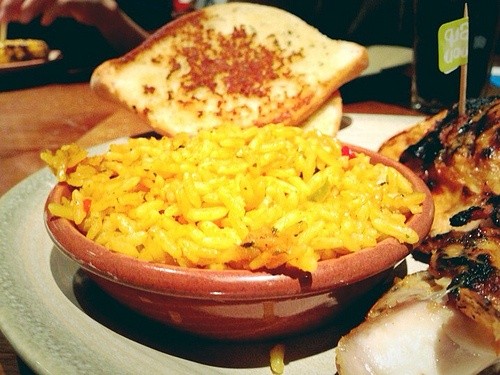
[[38, 124, 428, 273]]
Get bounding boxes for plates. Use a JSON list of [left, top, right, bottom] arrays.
[[0, 114, 431, 375]]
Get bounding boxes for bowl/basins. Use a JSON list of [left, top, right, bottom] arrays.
[[43, 129, 434, 339]]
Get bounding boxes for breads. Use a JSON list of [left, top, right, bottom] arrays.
[[90, 3, 370, 137]]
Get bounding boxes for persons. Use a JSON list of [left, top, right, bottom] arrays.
[[0, 0, 172, 82]]
